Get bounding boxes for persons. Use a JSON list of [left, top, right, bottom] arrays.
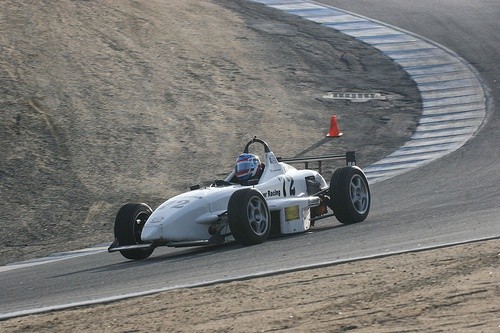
[[218, 153, 262, 187]]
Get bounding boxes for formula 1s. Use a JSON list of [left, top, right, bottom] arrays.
[[107, 136, 370, 260]]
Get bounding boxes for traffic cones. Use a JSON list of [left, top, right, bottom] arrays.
[[326, 115, 343, 137]]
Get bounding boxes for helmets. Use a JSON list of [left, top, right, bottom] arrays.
[[235, 153, 263, 185]]
